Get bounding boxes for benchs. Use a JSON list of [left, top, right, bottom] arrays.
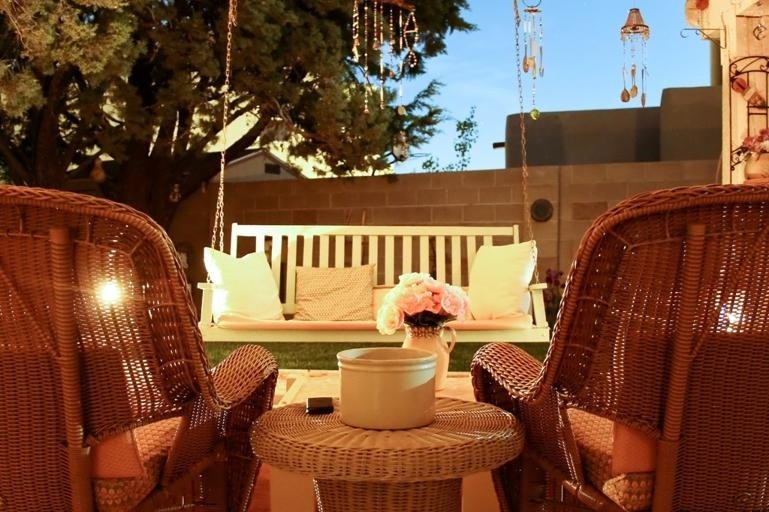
[[196, 223, 550, 342]]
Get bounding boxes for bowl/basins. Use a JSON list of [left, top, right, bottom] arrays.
[[337, 347, 437, 432]]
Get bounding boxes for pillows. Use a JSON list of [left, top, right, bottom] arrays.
[[295, 264, 377, 322], [468, 241, 538, 320], [205, 247, 286, 325]]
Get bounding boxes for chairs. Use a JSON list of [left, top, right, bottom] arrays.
[[34, 188, 277, 512], [470, 184, 747, 512]]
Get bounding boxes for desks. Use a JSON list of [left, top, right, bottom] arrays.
[[251, 396, 524, 512]]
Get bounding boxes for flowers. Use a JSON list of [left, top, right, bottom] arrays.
[[376, 273, 468, 335], [742, 128, 769, 153]]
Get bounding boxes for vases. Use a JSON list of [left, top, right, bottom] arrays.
[[402, 323, 456, 390], [745, 153, 769, 180]]
